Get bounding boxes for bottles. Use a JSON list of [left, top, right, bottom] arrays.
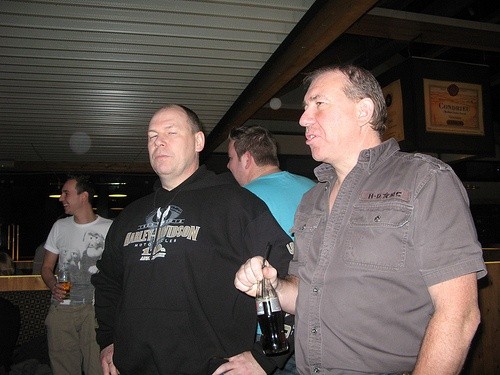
[[255, 264, 289, 357]]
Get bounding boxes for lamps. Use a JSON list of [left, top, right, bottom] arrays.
[[107, 185, 128, 198]]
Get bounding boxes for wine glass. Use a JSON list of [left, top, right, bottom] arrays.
[[57, 270, 71, 300]]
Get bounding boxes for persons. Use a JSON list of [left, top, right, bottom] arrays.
[[235, 60, 487, 375], [225, 124, 318, 243], [41, 173, 114, 375], [0, 252, 15, 276], [90, 104, 298, 375]]
